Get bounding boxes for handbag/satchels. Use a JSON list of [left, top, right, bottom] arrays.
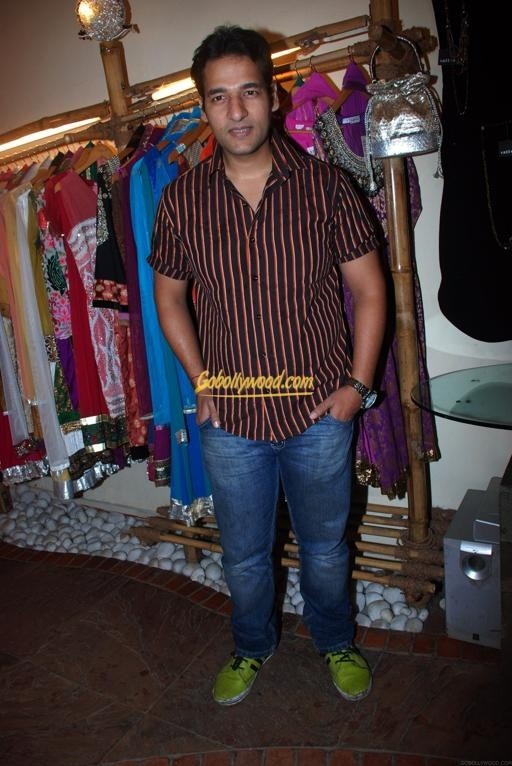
[[364, 34, 443, 160]]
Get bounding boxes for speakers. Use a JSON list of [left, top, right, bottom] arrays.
[[443, 487, 501, 650]]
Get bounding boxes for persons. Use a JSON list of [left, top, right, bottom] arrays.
[[149, 20, 386, 705]]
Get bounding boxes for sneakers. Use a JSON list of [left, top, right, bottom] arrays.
[[212, 647, 277, 706], [322, 645, 374, 703]]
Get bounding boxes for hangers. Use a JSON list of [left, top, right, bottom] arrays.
[[0, 125, 114, 197], [97, 42, 370, 181]]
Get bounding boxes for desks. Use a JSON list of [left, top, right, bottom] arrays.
[[411, 362, 510, 431]]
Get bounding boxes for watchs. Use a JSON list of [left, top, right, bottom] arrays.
[[345, 377, 380, 409]]
